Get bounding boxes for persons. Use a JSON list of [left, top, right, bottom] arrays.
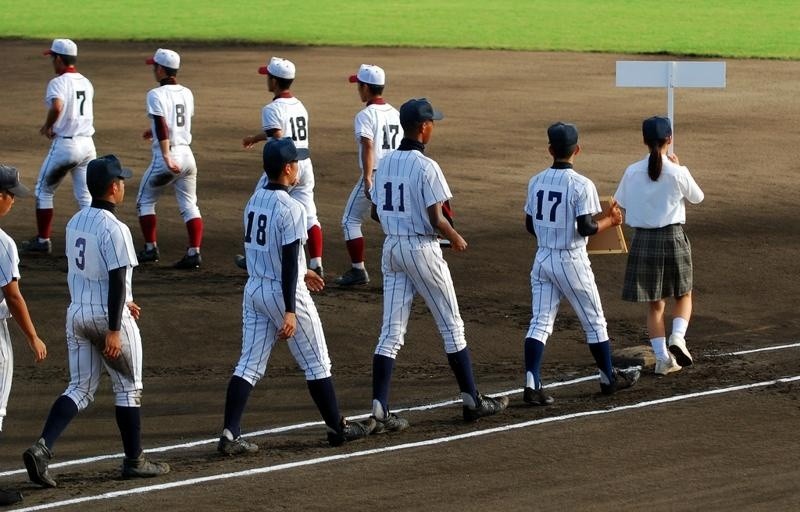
[[217, 138, 377, 456], [333, 63, 405, 285], [235, 57, 323, 280], [23, 155, 171, 487], [371, 98, 509, 434], [615, 116, 705, 376], [135, 48, 203, 269], [0, 164, 47, 430], [21, 39, 97, 255], [523, 122, 640, 405]]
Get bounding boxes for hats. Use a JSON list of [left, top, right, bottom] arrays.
[[263, 139, 310, 164], [398, 99, 443, 126], [641, 116, 672, 138], [0, 165, 32, 198], [86, 155, 132, 183], [544, 122, 578, 148], [257, 57, 295, 79], [145, 48, 181, 70], [349, 64, 386, 87], [42, 38, 78, 57]]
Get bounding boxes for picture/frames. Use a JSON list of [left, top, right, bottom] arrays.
[[586, 196, 629, 255]]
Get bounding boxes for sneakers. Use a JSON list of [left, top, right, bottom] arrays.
[[173, 253, 202, 269], [326, 416, 377, 447], [655, 356, 681, 376], [121, 448, 171, 479], [462, 394, 511, 423], [333, 264, 370, 287], [18, 236, 52, 256], [668, 334, 694, 368], [234, 254, 246, 270], [523, 384, 554, 406], [22, 437, 58, 487], [217, 435, 259, 455], [600, 366, 642, 396], [137, 244, 160, 262], [370, 413, 408, 437], [2, 487, 21, 505]]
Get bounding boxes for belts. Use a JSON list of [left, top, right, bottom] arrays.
[[62, 135, 71, 140]]
